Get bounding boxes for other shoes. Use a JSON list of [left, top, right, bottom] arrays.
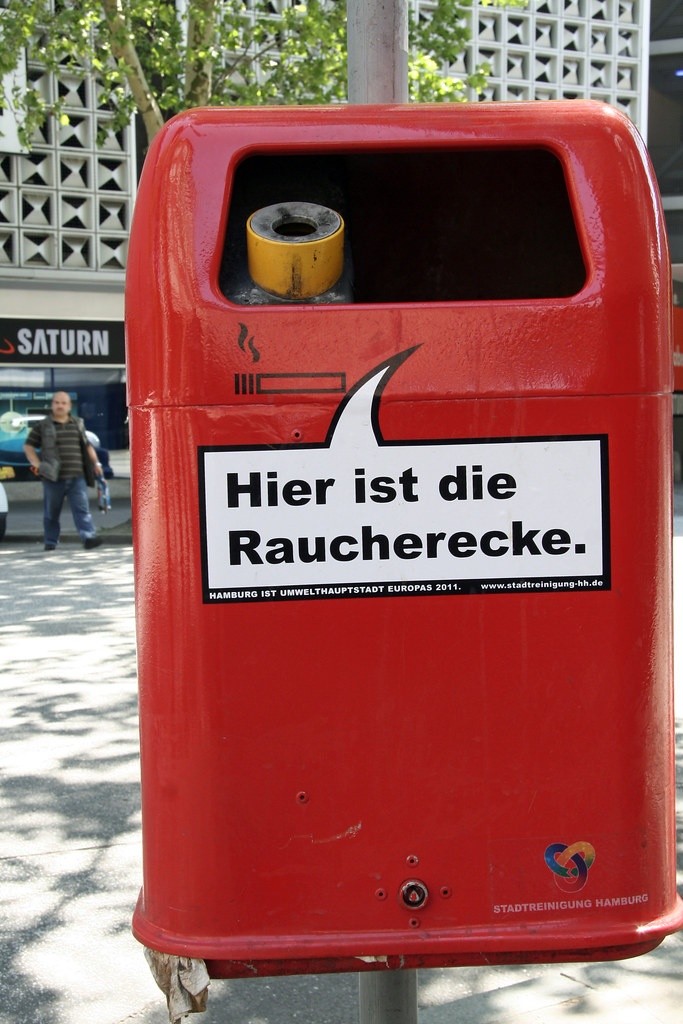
[[85, 536, 103, 549], [45, 544, 54, 550]]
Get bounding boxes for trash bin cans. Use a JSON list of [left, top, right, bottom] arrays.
[[129, 100, 683, 978]]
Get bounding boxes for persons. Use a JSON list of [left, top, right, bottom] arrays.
[[23, 392, 104, 550]]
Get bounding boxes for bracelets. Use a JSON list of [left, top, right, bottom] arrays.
[[94, 463, 102, 467]]
